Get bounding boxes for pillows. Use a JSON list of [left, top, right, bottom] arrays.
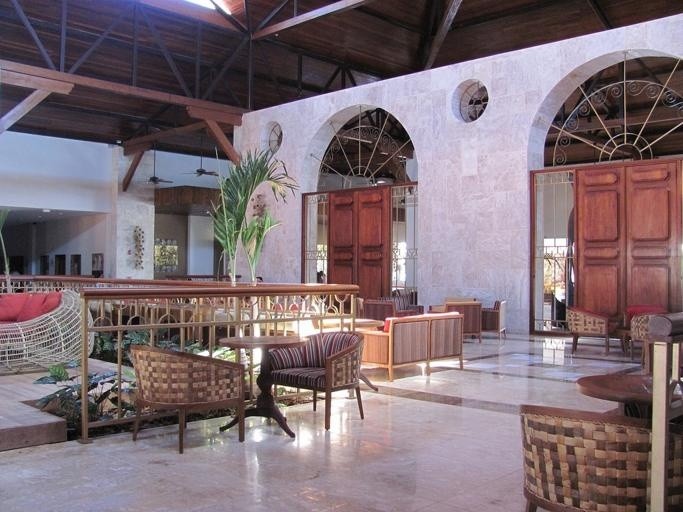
[[0, 291, 61, 322]]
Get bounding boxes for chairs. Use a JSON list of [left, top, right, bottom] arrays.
[[356, 298, 508, 344], [520, 305, 683, 512], [127, 331, 364, 454]]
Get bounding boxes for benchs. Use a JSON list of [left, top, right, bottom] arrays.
[[354, 310, 465, 382]]
